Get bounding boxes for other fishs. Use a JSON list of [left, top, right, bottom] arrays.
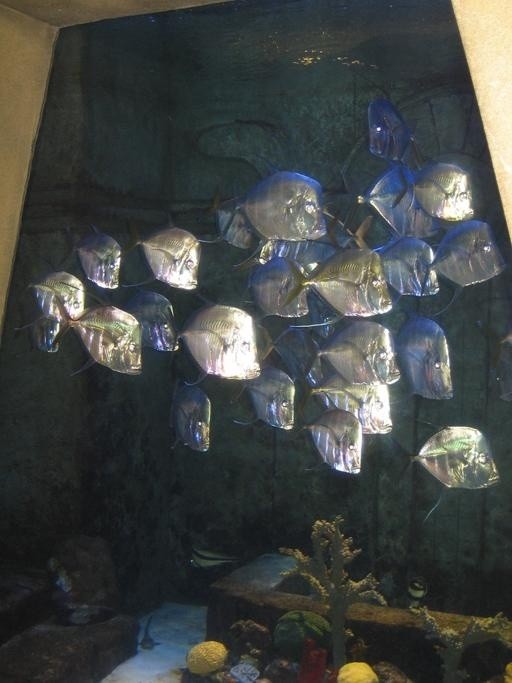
[[173, 153, 505, 450], [24, 220, 202, 375]]
